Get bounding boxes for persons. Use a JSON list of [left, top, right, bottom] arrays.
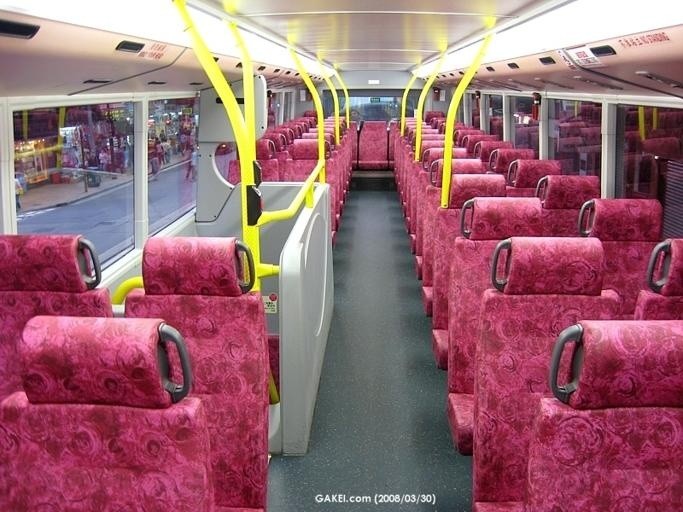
[[122, 146, 129, 168], [180, 132, 186, 157], [72, 145, 81, 168], [186, 133, 194, 152], [162, 139, 171, 163], [14, 174, 23, 211], [186, 147, 196, 181], [159, 130, 166, 141], [155, 141, 164, 165], [99, 148, 108, 171]]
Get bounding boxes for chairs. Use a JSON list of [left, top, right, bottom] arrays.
[[445, 196, 544, 457], [228, 138, 281, 186], [534, 174, 601, 238], [124, 235, 269, 512], [526, 318, 683, 512], [631, 237, 683, 322], [426, 108, 446, 124], [283, 138, 337, 251], [389, 118, 451, 146], [431, 173, 507, 371], [263, 133, 288, 182], [415, 146, 469, 280], [388, 117, 398, 169], [406, 147, 469, 257], [0, 233, 114, 406], [0, 315, 217, 512], [505, 158, 563, 198], [462, 133, 501, 158], [272, 127, 295, 161], [428, 118, 468, 134], [274, 115, 351, 197], [484, 148, 536, 186], [304, 108, 318, 118], [470, 235, 625, 511], [422, 157, 485, 318], [577, 198, 664, 320], [473, 141, 515, 174], [455, 131, 484, 144], [358, 120, 388, 169]]
